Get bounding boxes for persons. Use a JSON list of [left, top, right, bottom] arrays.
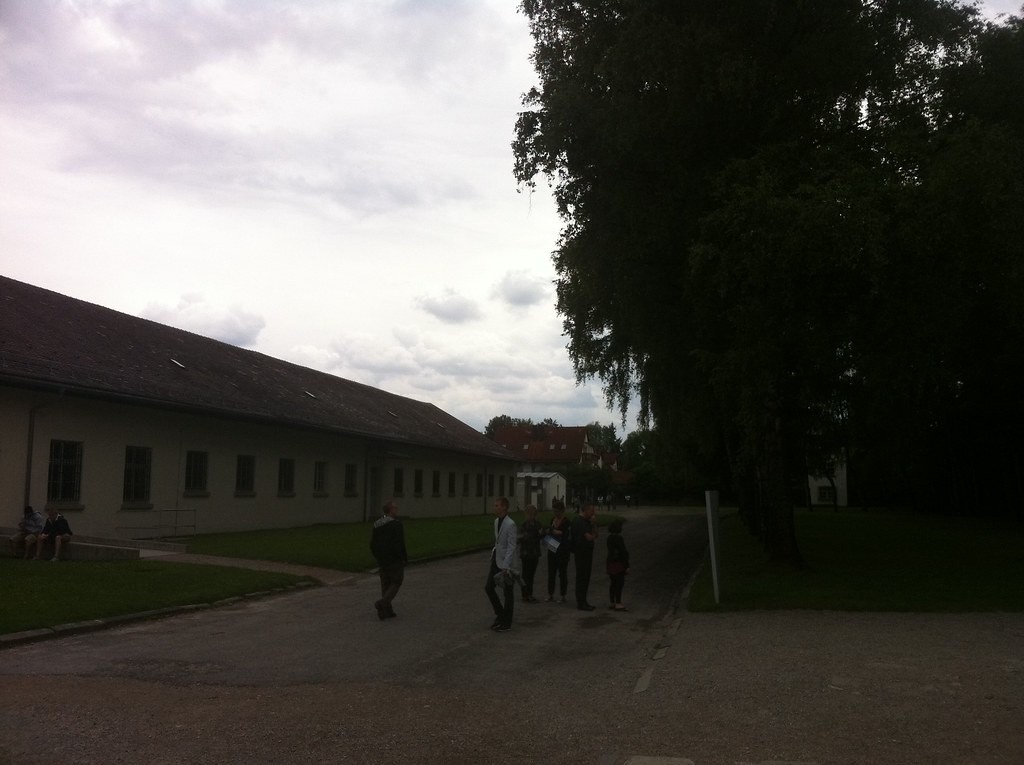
[[485, 496, 521, 633], [570, 502, 599, 612], [598, 493, 604, 511], [369, 501, 408, 620], [518, 505, 543, 602], [7, 506, 44, 561], [33, 507, 72, 564], [544, 499, 571, 604], [605, 520, 631, 611], [607, 492, 618, 511]]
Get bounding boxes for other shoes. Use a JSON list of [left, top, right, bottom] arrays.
[[556, 596, 567, 604], [491, 621, 502, 630], [376, 599, 388, 621], [614, 603, 625, 610], [387, 611, 397, 617], [497, 624, 511, 632], [543, 596, 553, 602], [521, 595, 537, 603], [577, 604, 595, 611], [609, 602, 615, 608]]
